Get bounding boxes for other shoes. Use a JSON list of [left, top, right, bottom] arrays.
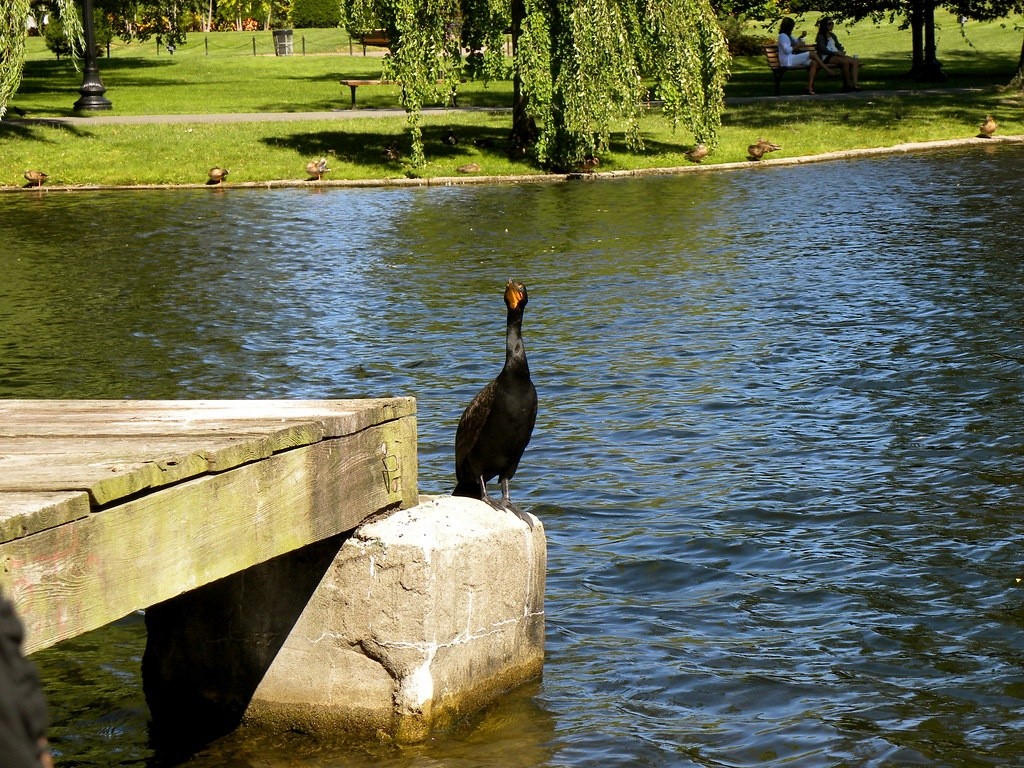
[[843, 79, 861, 92], [808, 88, 815, 94], [828, 71, 836, 76]]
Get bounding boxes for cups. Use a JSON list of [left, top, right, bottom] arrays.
[[800, 37, 806, 45], [852, 54, 860, 60]]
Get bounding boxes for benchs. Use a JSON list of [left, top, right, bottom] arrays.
[[340, 77, 467, 109], [764, 46, 842, 95]]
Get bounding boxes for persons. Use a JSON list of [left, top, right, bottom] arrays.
[[777, 17, 840, 95], [815, 17, 862, 92]]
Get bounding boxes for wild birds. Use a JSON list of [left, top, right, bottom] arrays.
[[575, 154, 599, 167], [455, 162, 481, 173], [978, 114, 999, 138], [208, 166, 228, 186], [305, 157, 332, 181], [451, 278, 537, 532], [439, 127, 458, 145], [748, 137, 781, 161], [382, 143, 402, 161], [684, 143, 708, 165], [24, 169, 50, 188]]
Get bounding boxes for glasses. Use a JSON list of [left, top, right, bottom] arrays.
[[827, 22, 833, 26]]
